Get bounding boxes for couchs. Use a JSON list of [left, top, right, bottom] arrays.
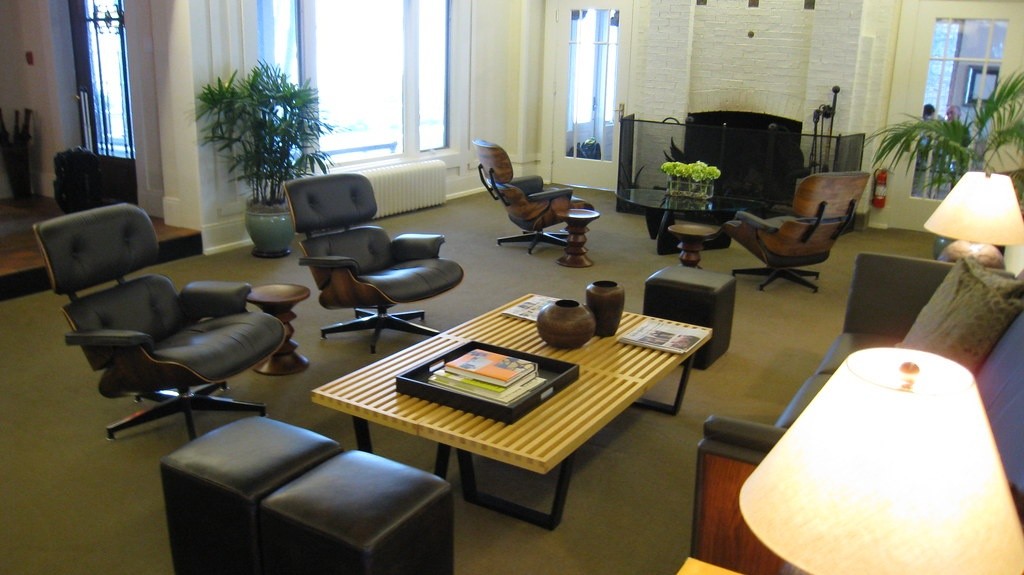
[[691, 253, 1024, 575]]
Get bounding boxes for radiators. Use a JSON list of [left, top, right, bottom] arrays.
[[352, 159, 448, 220]]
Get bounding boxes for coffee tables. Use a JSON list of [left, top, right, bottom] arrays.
[[310, 294, 714, 527]]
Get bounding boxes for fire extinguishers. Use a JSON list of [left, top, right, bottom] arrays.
[[872, 168, 887, 208]]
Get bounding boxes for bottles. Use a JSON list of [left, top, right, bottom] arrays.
[[586, 280, 625, 337], [537, 299, 596, 347]]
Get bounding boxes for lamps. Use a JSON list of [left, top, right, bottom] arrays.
[[923, 167, 1024, 271], [738, 349, 1024, 575]]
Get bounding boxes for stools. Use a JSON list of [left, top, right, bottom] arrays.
[[667, 224, 716, 268], [555, 206, 600, 268], [246, 284, 310, 376], [642, 266, 737, 371]]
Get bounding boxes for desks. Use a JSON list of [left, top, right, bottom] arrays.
[[613, 188, 747, 256]]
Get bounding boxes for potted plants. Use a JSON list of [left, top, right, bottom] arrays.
[[860, 62, 1024, 262], [185, 57, 352, 258], [660, 161, 721, 200]]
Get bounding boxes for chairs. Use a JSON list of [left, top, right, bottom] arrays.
[[30, 202, 287, 443], [729, 171, 871, 293], [280, 174, 464, 356], [471, 138, 595, 256]]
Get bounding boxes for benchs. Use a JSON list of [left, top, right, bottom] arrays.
[[157, 415, 456, 575]]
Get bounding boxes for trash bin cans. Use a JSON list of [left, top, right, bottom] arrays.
[[54, 147, 104, 213]]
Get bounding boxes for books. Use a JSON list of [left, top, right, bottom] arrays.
[[617, 320, 709, 355], [500, 297, 557, 322], [428, 348, 547, 406]]
